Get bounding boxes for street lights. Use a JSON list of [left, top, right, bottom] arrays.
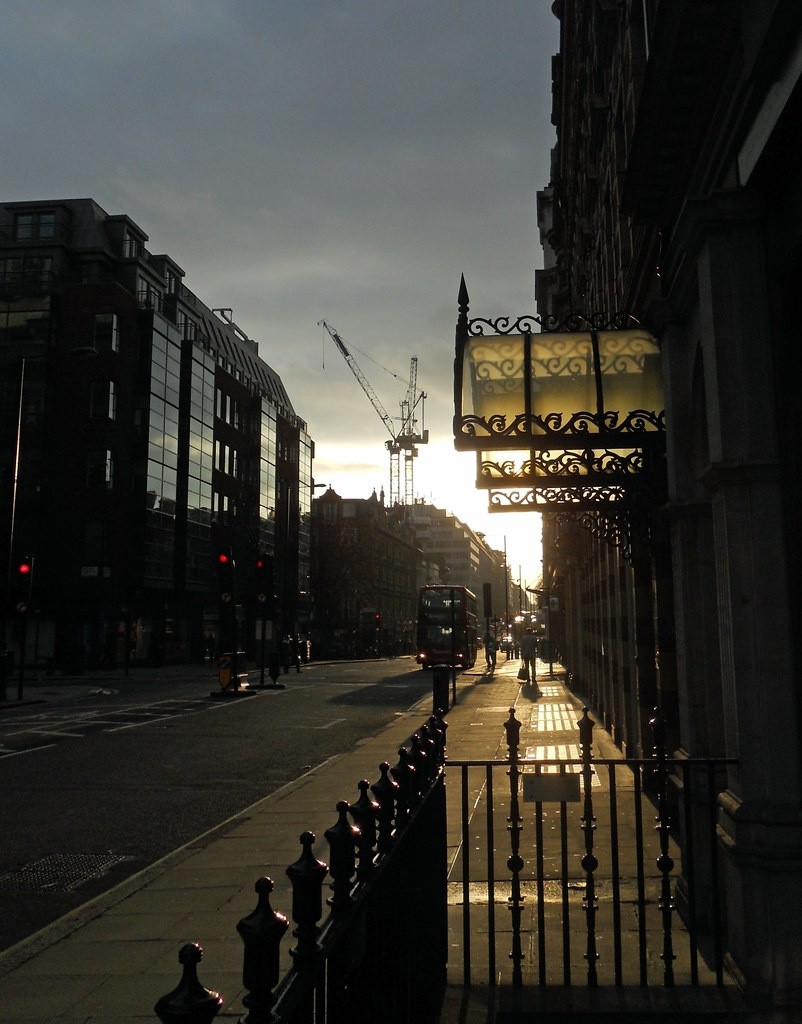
[[283, 483, 326, 638], [7, 347, 100, 618]]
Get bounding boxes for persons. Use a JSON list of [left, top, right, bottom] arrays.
[[483, 626, 499, 674], [283, 634, 304, 674], [521, 628, 538, 682]]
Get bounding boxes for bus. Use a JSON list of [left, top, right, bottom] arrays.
[[415, 585, 478, 670]]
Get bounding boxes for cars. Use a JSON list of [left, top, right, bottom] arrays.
[[500, 637, 515, 651], [476, 637, 483, 650]]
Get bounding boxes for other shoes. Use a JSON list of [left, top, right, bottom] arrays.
[[532, 676, 536, 682], [486, 664, 492, 669], [526, 677, 530, 682], [492, 663, 495, 670], [296, 670, 303, 673], [284, 671, 290, 675]]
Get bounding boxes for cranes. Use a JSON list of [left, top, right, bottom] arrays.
[[317, 316, 428, 508]]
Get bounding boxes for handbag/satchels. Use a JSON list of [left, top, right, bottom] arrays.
[[518, 666, 526, 680]]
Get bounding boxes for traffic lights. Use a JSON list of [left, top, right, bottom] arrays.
[[214, 545, 236, 605], [12, 555, 33, 619], [252, 554, 281, 619], [375, 613, 382, 632]]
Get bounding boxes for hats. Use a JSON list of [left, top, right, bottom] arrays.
[[484, 632, 490, 637]]
